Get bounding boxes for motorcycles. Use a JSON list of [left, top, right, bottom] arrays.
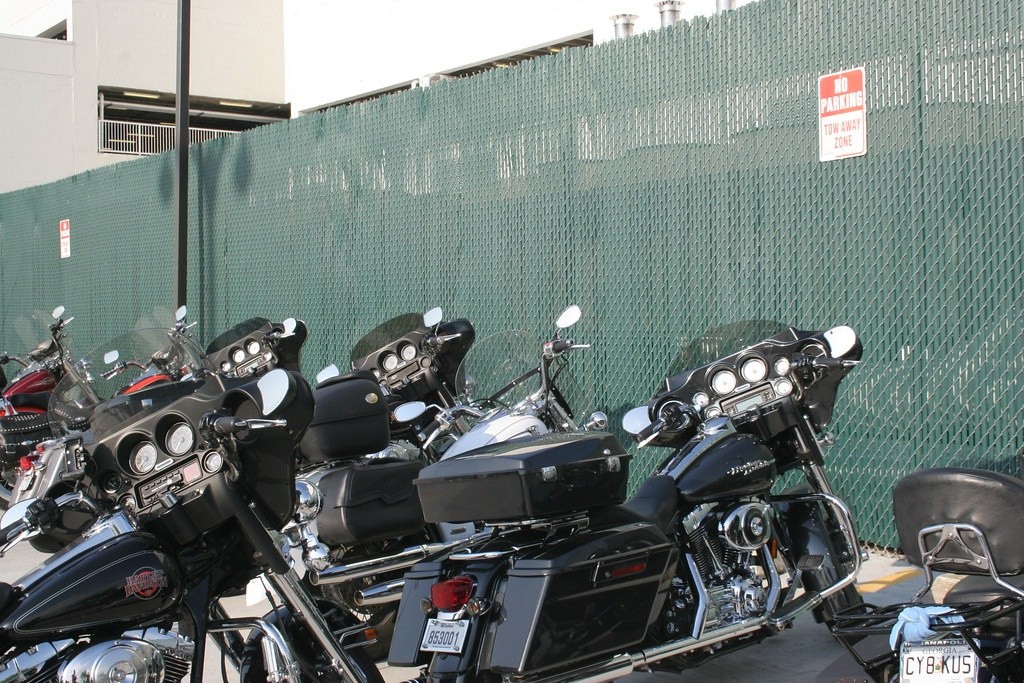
[[0, 290, 1024, 683]]
